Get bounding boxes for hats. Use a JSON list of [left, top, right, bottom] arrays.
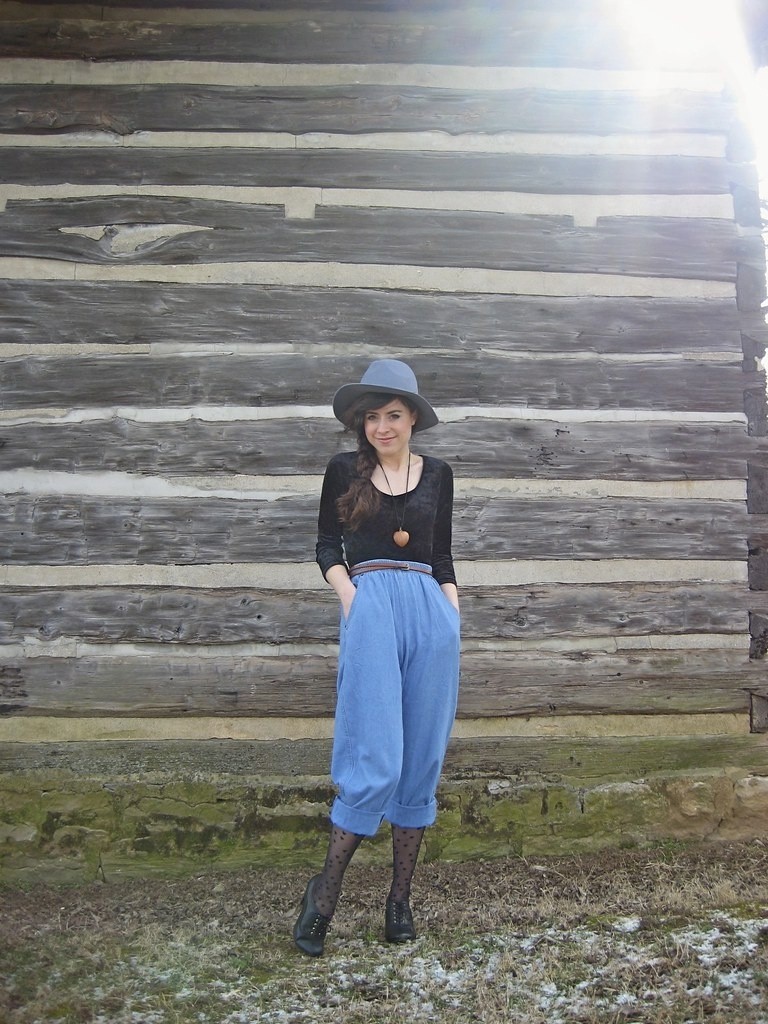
[[333, 359, 439, 435]]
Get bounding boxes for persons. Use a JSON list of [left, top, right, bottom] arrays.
[[295, 358, 460, 958]]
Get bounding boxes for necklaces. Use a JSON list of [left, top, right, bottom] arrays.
[[376, 452, 410, 547]]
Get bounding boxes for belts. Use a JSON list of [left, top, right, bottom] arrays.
[[348, 562, 433, 580]]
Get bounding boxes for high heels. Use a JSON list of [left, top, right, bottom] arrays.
[[292, 873, 332, 956], [385, 898, 416, 943]]
